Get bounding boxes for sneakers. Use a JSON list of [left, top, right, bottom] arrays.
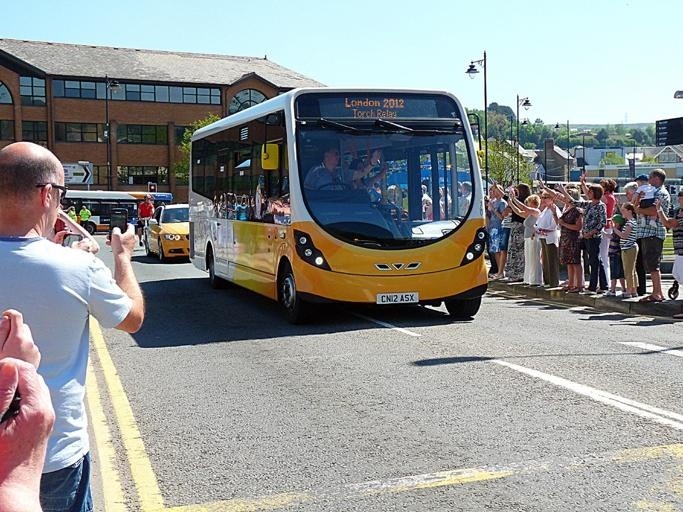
[[649, 220, 657, 228], [488, 270, 646, 298]]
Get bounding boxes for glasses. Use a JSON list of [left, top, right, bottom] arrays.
[[35, 183, 66, 199]]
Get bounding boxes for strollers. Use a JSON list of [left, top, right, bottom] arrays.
[[667, 256, 682, 299]]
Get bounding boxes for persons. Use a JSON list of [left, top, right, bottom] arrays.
[[210, 193, 298, 225], [2, 357, 55, 511], [135, 195, 154, 247], [0, 141, 146, 511], [300, 142, 393, 205], [415, 170, 683, 303], [55, 203, 100, 254], [0, 308, 45, 375]]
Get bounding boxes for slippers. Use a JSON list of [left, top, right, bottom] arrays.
[[639, 296, 662, 303]]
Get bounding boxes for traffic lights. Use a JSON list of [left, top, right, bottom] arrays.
[[148, 183, 156, 193]]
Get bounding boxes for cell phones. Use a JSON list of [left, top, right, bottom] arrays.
[[109, 208, 128, 241]]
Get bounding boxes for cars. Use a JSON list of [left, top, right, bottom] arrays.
[[193, 192, 291, 250], [400, 183, 407, 194], [143, 203, 188, 261]]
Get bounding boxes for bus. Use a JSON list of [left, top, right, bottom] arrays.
[[187, 88, 488, 327], [59, 189, 172, 236]]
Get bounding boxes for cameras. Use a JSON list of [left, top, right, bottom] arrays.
[[63, 233, 83, 248], [485, 196, 489, 205]]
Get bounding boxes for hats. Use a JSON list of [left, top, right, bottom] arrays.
[[613, 214, 624, 226], [634, 175, 648, 182]]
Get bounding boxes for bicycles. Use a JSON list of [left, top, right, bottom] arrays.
[[135, 216, 153, 247]]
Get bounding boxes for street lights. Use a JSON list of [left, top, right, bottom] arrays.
[[516, 94, 532, 186], [104, 73, 120, 191], [624, 139, 636, 178], [466, 49, 486, 199], [567, 146, 584, 182], [574, 131, 584, 171], [553, 120, 569, 183], [510, 117, 527, 181]]
